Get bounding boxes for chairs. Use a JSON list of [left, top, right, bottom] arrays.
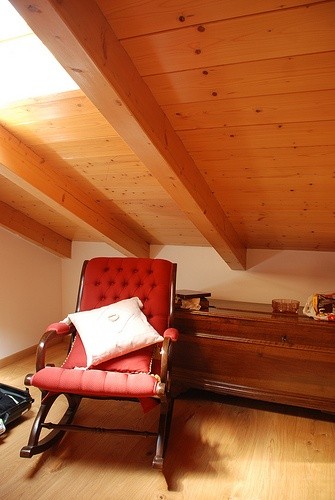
[[20, 257, 180, 471]]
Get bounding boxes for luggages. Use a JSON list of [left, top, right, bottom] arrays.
[[0, 382, 34, 425]]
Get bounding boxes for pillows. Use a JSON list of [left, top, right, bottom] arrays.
[[67, 297, 164, 370]]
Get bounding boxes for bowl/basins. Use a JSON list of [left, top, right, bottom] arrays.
[[272, 299, 300, 312]]
